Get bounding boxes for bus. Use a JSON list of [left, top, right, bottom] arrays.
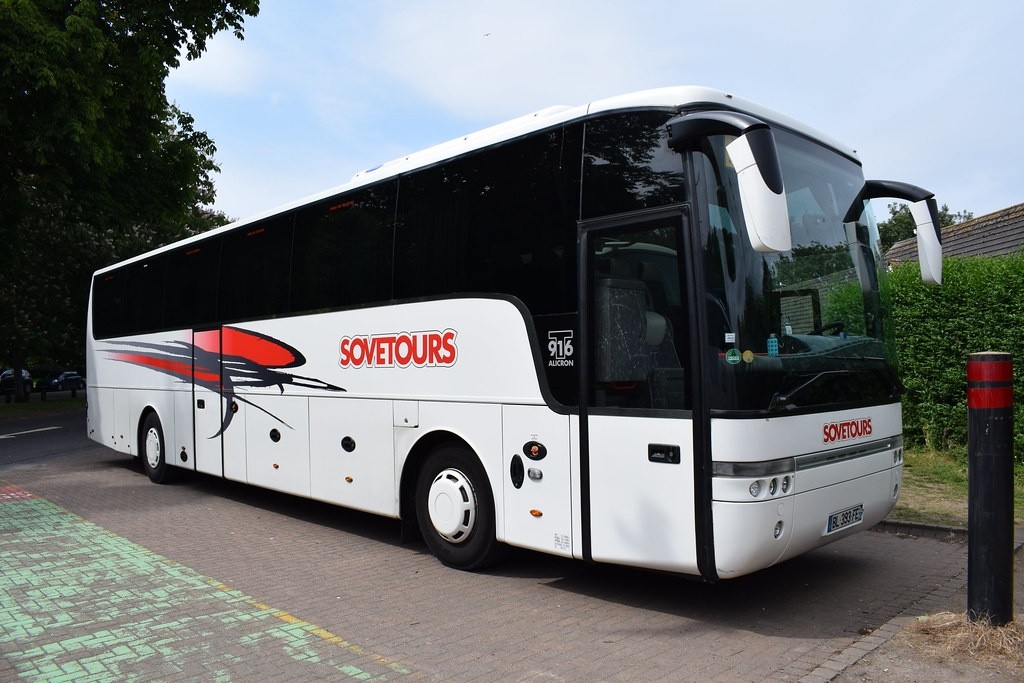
[[83, 86, 945, 587]]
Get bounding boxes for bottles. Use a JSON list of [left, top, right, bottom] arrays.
[[767, 334, 778, 356]]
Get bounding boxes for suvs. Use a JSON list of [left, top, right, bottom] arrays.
[[0, 368, 34, 392]]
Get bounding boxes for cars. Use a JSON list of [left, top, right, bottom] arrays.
[[57, 371, 81, 391]]
